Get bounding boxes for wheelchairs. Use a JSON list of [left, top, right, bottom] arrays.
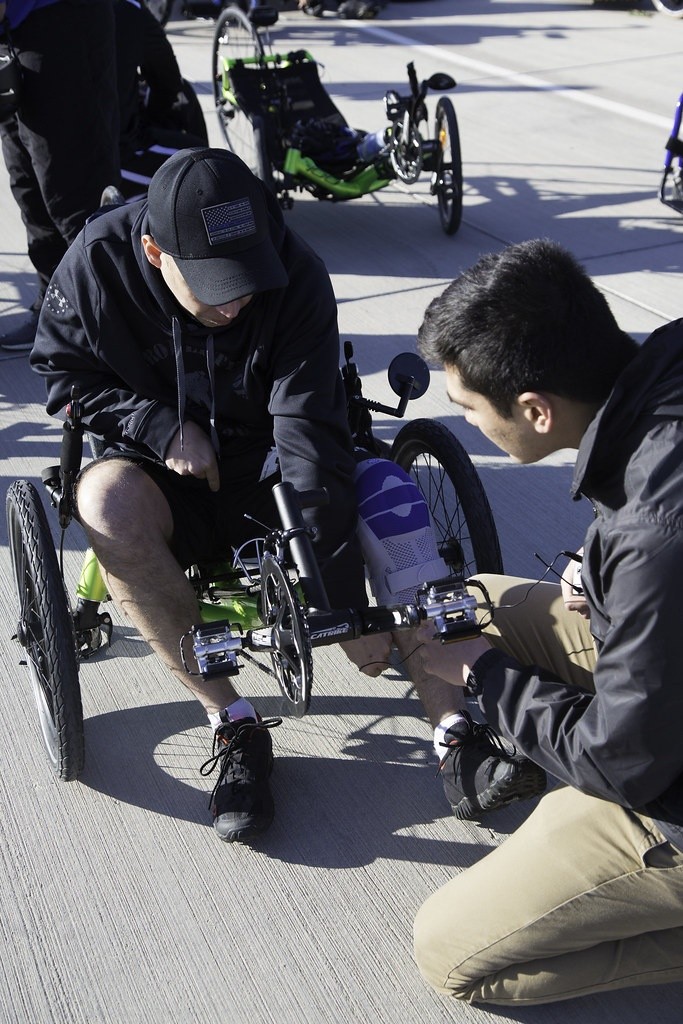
[[5, 343, 550, 802]]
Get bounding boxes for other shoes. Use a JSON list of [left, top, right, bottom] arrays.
[[1, 312, 41, 350]]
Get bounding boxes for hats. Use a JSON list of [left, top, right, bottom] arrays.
[[147, 147, 289, 305]]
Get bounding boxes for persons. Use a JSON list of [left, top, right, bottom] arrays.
[[0, 0, 549, 842], [413, 239, 683, 1005]]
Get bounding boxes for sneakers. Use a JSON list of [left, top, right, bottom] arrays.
[[200, 708, 283, 843], [435, 709, 547, 821]]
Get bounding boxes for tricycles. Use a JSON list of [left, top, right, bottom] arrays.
[[209, 5, 464, 233]]
